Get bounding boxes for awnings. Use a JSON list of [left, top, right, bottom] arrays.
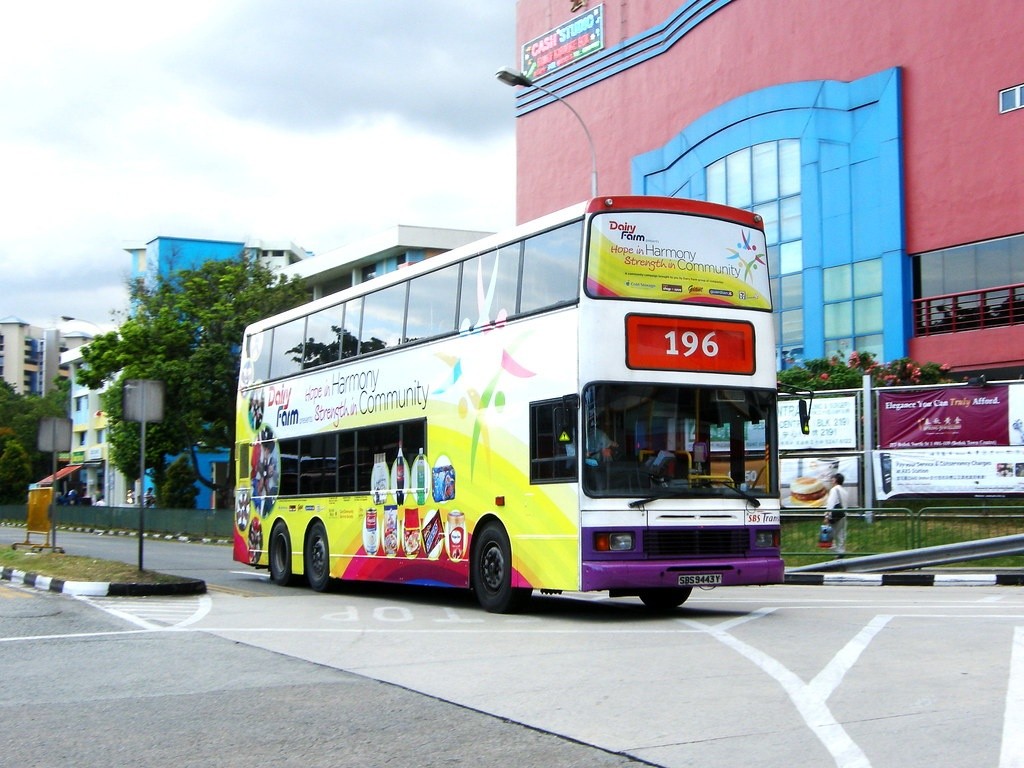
[[37, 461, 101, 485]]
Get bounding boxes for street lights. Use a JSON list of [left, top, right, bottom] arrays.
[[61, 315, 110, 506], [496, 68, 597, 197]]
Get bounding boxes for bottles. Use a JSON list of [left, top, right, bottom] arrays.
[[396, 441, 405, 505], [418, 447, 426, 505]]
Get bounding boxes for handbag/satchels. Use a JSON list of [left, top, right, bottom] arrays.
[[818, 521, 833, 548], [828, 488, 845, 525]]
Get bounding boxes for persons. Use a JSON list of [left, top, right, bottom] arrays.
[[823, 474, 848, 559], [144, 487, 156, 508], [566, 414, 619, 470]]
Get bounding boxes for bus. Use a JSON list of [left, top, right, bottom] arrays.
[[233, 196, 814, 613]]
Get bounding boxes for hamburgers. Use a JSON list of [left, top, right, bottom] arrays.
[[789, 477, 828, 507]]
